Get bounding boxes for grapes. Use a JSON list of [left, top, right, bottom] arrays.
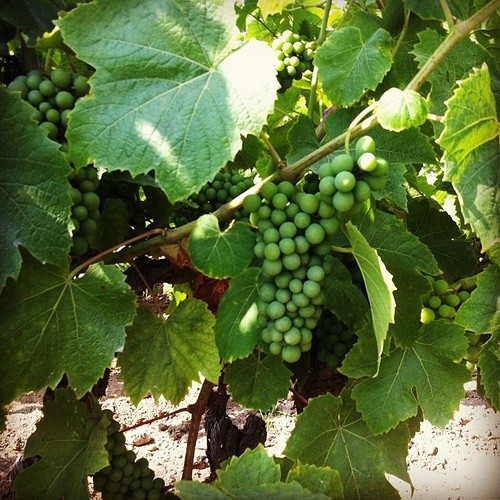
[[7, 31, 486, 500]]
